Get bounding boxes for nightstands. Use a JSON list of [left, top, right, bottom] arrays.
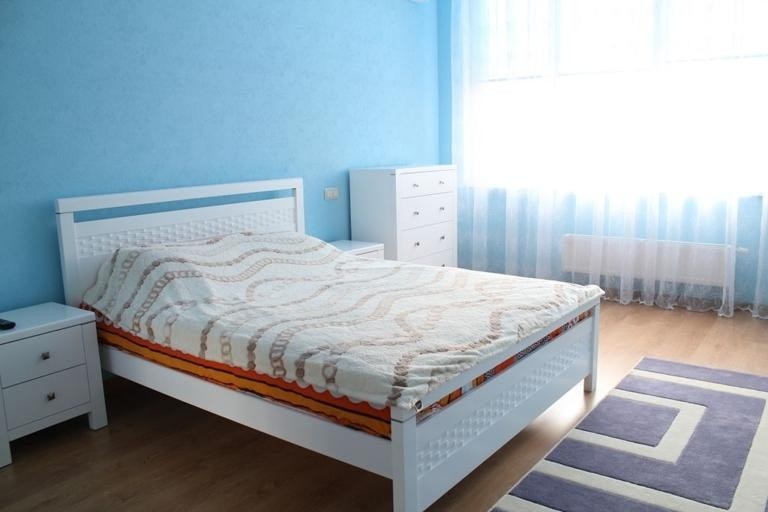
[[1, 302, 109, 471], [329, 239, 385, 261]]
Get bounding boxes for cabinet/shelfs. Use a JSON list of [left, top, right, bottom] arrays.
[[349, 164, 460, 266]]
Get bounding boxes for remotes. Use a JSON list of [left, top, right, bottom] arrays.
[[0, 319, 15, 330]]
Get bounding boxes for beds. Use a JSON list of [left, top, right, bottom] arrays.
[[54, 175, 606, 512]]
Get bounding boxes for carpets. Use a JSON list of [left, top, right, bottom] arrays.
[[484, 355, 768, 511]]
